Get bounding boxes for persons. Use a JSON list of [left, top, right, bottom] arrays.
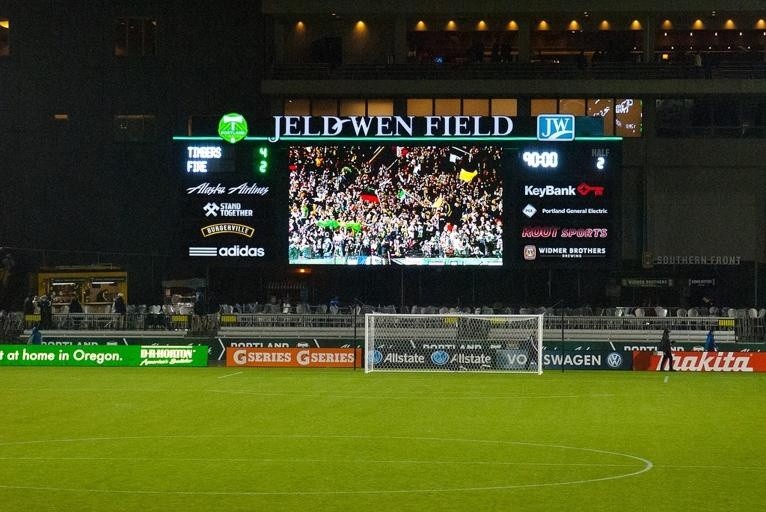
[[659, 328, 677, 372], [287, 145, 503, 259], [704, 328, 719, 351], [1, 286, 222, 346]]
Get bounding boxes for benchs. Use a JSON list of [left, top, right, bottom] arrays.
[[426, 42, 766, 79]]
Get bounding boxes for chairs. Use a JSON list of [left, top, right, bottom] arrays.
[[0, 298, 766, 346]]
